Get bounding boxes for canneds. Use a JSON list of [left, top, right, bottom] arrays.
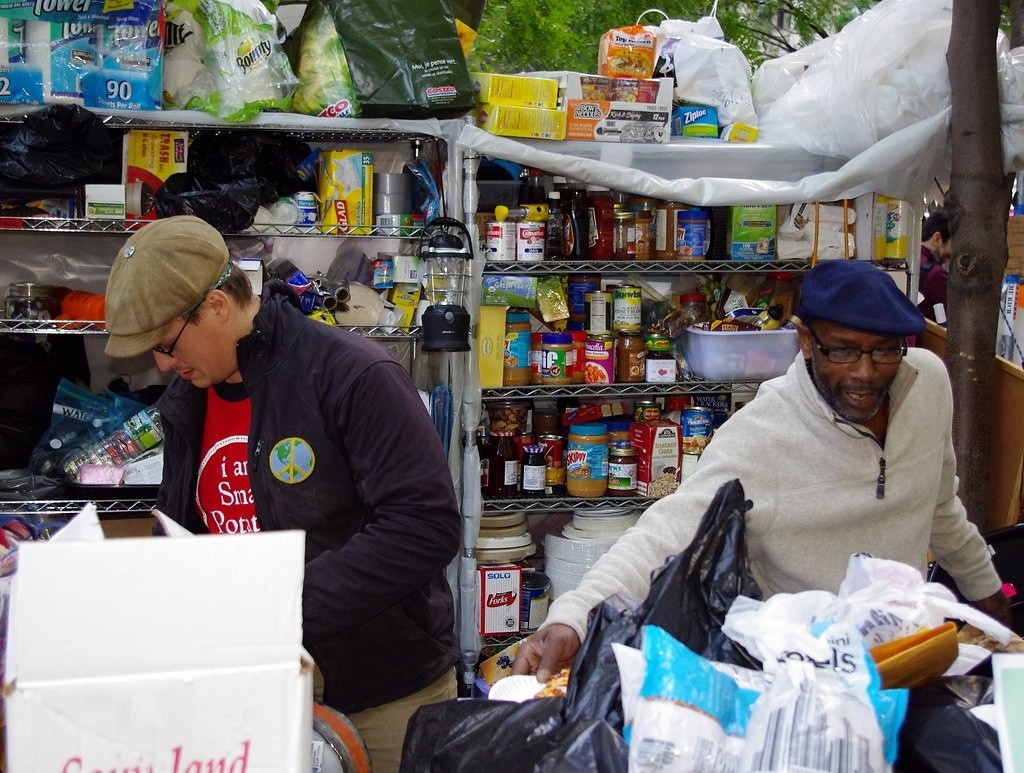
[[502, 267, 706, 384], [754, 271, 801, 332], [473, 402, 716, 499], [520, 571, 551, 631], [484, 204, 708, 262]]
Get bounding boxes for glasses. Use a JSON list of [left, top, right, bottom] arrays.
[[152, 286, 223, 358], [804, 323, 908, 365]]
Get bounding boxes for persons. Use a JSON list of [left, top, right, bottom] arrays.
[[916, 212, 951, 323], [510, 258, 1019, 682], [102, 214, 463, 772]]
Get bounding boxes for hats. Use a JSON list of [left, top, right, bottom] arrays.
[[800, 261, 926, 336], [104, 215, 229, 358]]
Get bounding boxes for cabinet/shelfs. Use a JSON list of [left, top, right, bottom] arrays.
[[0, 110, 454, 515], [461, 113, 921, 698]]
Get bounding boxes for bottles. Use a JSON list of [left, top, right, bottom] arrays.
[[4, 281, 56, 329], [526, 168, 612, 260], [681, 292, 707, 324], [61, 404, 165, 484]]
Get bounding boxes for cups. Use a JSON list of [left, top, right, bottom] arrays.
[[372, 172, 412, 215], [54, 289, 107, 329]]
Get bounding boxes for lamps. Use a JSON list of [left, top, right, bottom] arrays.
[[417, 215, 475, 353]]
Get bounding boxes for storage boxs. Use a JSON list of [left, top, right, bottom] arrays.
[[4, 500, 315, 773], [393, 304, 415, 333], [478, 304, 507, 388], [561, 401, 626, 427], [388, 282, 422, 308], [124, 452, 164, 484], [121, 129, 189, 231], [628, 418, 683, 498], [680, 326, 799, 379], [730, 204, 776, 260], [317, 148, 374, 236], [233, 256, 267, 296], [478, 634, 534, 685], [377, 250, 420, 283], [479, 273, 538, 308], [478, 179, 523, 208], [475, 563, 521, 636], [84, 183, 126, 219], [375, 212, 426, 237], [468, 69, 760, 144]]
